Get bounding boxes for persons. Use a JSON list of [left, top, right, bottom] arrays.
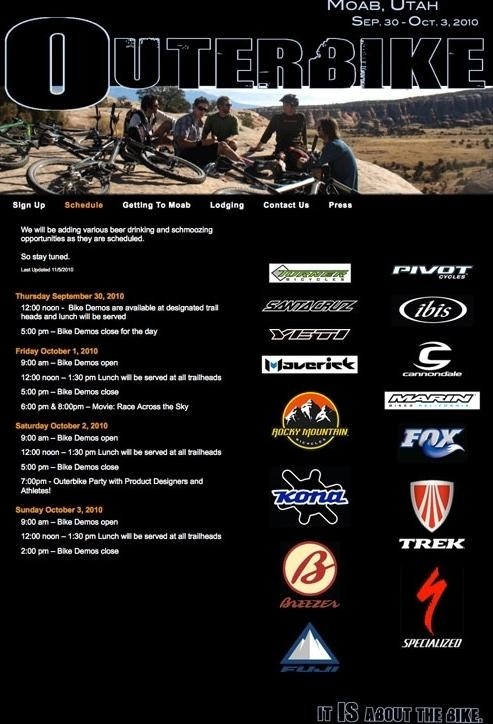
[[124, 93, 359, 195]]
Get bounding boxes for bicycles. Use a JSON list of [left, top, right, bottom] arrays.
[[211, 141, 360, 195], [0, 113, 207, 196]]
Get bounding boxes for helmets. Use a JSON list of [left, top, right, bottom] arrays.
[[204, 162, 225, 177], [281, 94, 299, 106]]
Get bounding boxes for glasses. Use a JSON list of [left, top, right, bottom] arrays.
[[196, 106, 209, 112]]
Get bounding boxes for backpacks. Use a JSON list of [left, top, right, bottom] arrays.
[[124, 109, 148, 136]]
